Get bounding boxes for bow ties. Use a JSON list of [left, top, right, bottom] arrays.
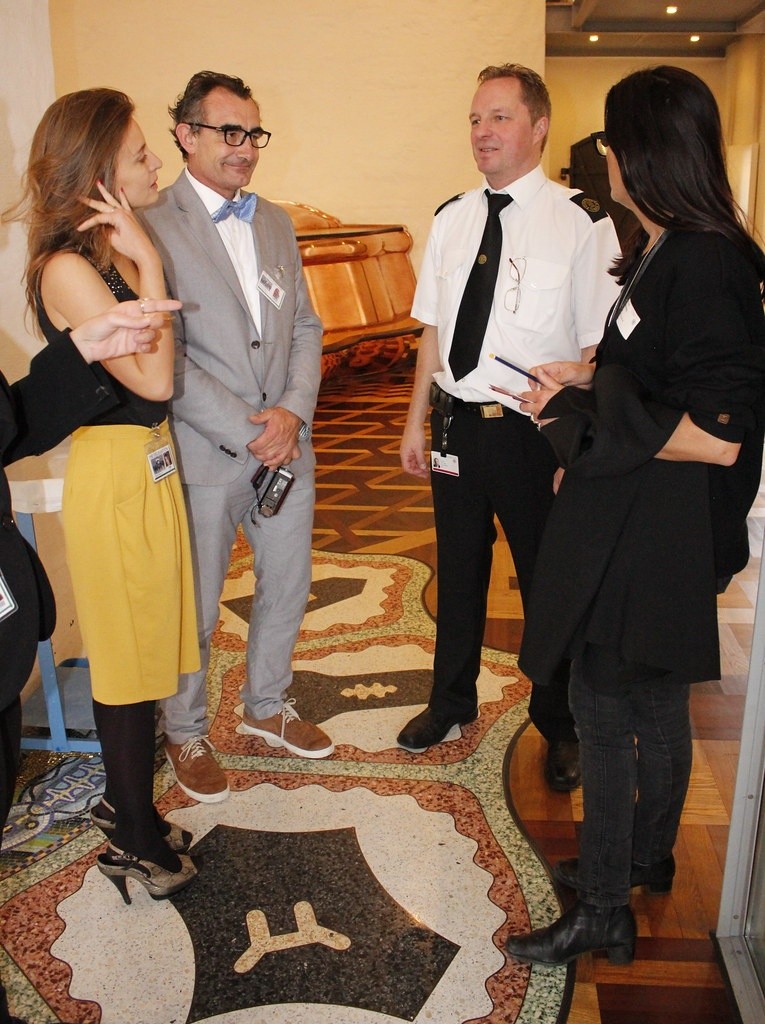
[[210, 193, 258, 224]]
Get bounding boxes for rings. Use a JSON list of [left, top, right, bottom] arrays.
[[111, 206, 118, 213], [536, 423, 541, 432], [531, 413, 536, 422], [140, 298, 154, 314]]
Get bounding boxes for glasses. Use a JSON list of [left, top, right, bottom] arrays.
[[504, 256, 527, 314], [590, 131, 606, 159], [184, 122, 271, 148]]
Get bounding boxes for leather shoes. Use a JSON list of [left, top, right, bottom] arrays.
[[397, 707, 479, 749], [544, 727, 581, 791]]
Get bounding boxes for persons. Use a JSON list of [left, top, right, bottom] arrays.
[[505, 64, 765, 990], [162, 452, 172, 467], [399, 61, 626, 797], [0, 294, 182, 847], [125, 73, 338, 805], [434, 458, 440, 468], [19, 92, 206, 904]]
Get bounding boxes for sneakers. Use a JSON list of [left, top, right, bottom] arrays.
[[165, 734, 229, 803], [241, 698, 334, 757]]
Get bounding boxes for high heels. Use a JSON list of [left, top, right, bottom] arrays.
[[90, 797, 192, 853], [505, 900, 637, 966], [95, 839, 203, 906], [553, 853, 676, 893]]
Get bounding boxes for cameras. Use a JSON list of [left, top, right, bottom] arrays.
[[258, 466, 294, 518]]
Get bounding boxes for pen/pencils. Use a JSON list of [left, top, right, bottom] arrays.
[[489, 354, 543, 385]]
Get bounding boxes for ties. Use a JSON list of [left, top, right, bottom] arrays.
[[448, 193, 514, 382]]
